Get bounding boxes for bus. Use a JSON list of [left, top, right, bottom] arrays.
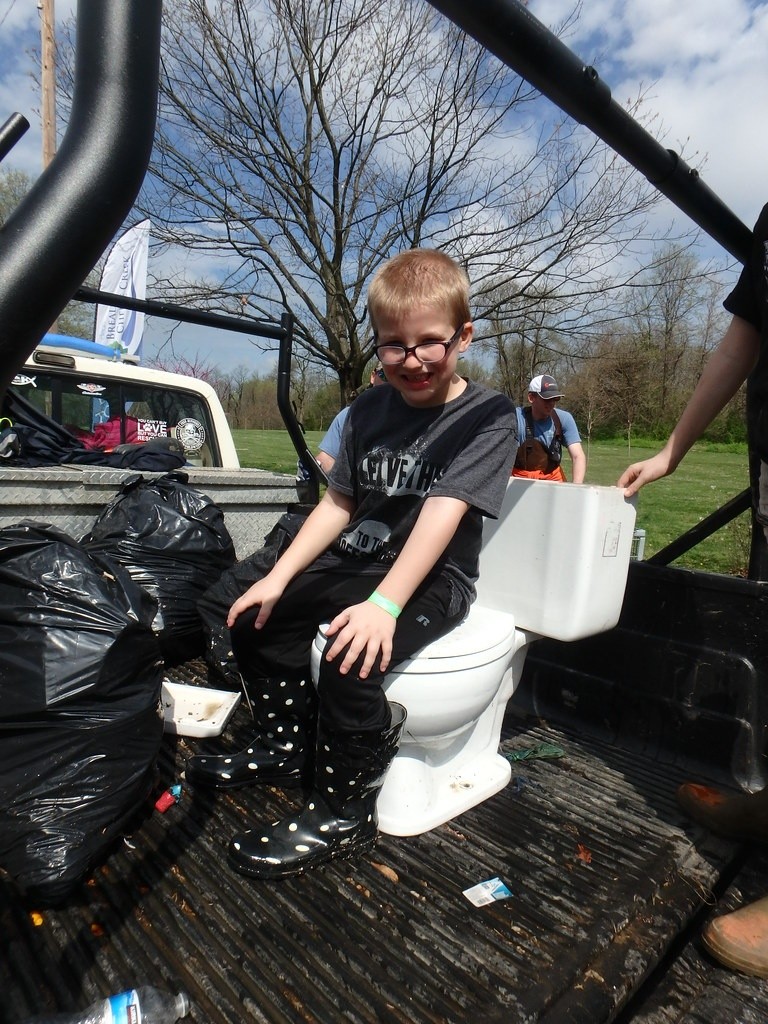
[[1, 334, 255, 485]]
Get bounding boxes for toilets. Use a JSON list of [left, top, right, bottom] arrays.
[[311, 475, 638, 838]]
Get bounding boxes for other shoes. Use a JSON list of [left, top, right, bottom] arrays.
[[701, 895, 768, 978], [678, 783, 768, 838]]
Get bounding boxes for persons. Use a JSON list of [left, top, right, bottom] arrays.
[[701, 884, 768, 992], [516, 374, 588, 484], [613, 200, 768, 572], [186, 247, 521, 882]]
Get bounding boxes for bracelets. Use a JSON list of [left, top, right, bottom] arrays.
[[365, 592, 403, 620]]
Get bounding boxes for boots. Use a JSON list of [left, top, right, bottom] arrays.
[[227, 701, 407, 877], [184, 674, 316, 789]]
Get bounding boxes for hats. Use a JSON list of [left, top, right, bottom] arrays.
[[374, 361, 383, 372], [529, 374, 565, 400]]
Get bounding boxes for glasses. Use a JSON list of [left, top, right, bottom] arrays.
[[377, 371, 387, 382], [534, 394, 560, 401], [373, 324, 464, 366]]
